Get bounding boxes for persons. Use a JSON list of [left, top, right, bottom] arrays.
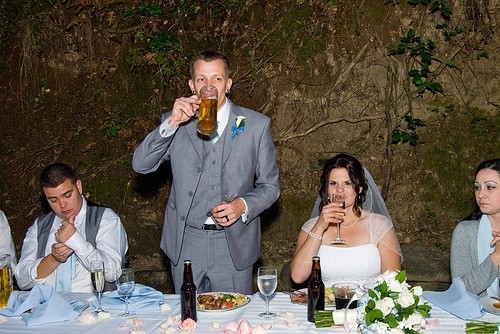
[[290, 154, 404, 289], [450, 159, 500, 300], [133, 50, 281, 295], [0, 163, 128, 294]]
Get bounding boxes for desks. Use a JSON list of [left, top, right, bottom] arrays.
[[0, 291, 500, 334]]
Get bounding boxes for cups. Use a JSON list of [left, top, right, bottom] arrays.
[[332, 283, 360, 309], [0, 254, 13, 309], [192, 85, 218, 135]]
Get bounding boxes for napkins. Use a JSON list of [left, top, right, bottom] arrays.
[[0, 282, 164, 326], [422, 278, 484, 321]]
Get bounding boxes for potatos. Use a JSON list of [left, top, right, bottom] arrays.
[[204, 299, 226, 310]]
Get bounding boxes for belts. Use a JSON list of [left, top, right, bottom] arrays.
[[202, 224, 220, 232]]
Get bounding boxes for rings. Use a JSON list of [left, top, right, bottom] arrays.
[[225, 215, 230, 222], [61, 253, 64, 257]]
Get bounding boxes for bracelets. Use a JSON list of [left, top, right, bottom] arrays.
[[308, 232, 322, 241]]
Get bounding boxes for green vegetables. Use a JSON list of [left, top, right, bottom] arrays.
[[217, 293, 236, 302]]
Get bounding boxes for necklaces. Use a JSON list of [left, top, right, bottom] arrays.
[[339, 206, 362, 229]]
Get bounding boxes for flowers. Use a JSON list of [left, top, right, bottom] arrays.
[[230, 115, 247, 140], [314, 269, 441, 334]]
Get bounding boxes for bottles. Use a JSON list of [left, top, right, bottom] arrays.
[[307, 256, 325, 323], [180, 260, 197, 322]]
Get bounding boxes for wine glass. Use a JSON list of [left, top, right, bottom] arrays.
[[331, 193, 347, 244], [89, 261, 108, 314], [256, 266, 277, 318], [115, 268, 136, 317]]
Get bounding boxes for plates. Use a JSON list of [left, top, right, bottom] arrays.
[[479, 295, 500, 315], [297, 288, 335, 307]]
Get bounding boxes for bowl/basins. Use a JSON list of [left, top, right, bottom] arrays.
[[196, 292, 251, 323]]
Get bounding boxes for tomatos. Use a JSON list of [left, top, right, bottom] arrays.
[[226, 302, 232, 308]]
